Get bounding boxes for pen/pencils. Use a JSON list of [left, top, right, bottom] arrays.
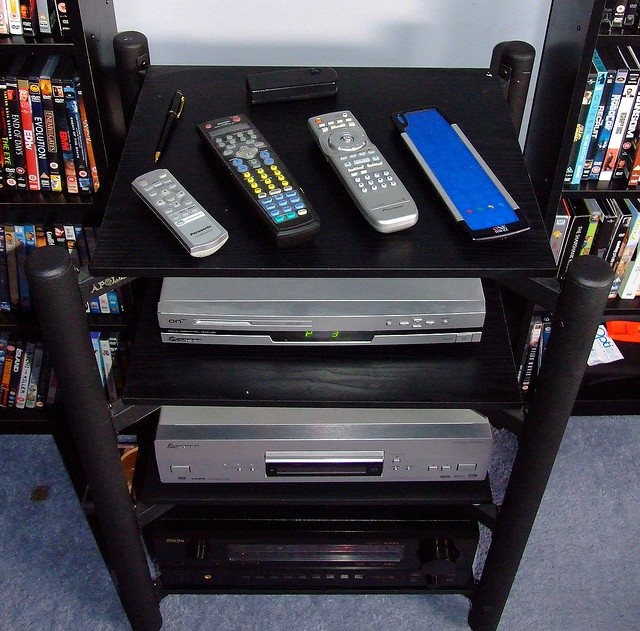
[[155, 90, 185, 163]]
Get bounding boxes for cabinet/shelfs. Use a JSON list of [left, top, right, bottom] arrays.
[[20, 28, 615, 631], [524, 1, 640, 415], [0, 2, 121, 434]]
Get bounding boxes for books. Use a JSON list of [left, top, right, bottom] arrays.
[[549, 198, 640, 302], [518, 316, 552, 394], [0, 332, 129, 412], [0, 0, 72, 37], [563, 46, 640, 186], [599, 0, 640, 37], [0, 55, 100, 195], [0, 212, 126, 315]]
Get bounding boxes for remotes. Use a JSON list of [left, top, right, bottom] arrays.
[[196, 111, 321, 241], [131, 168, 231, 259], [246, 66, 340, 107], [308, 110, 420, 233]]
